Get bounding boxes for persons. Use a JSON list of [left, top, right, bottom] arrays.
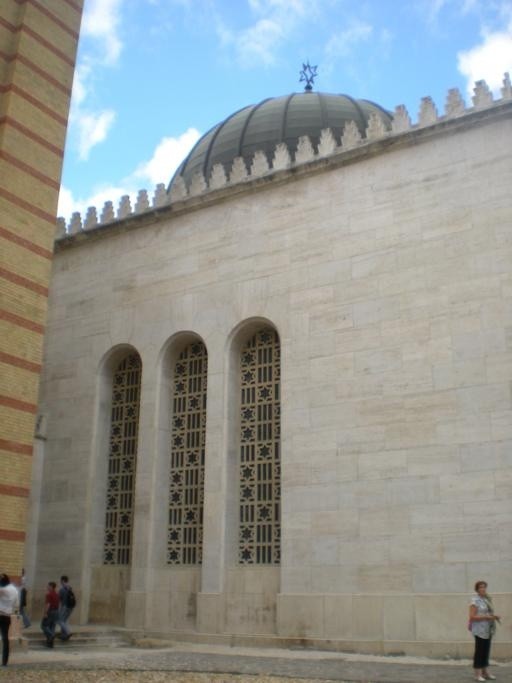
[[56, 575, 77, 640], [20, 568, 32, 628], [41, 581, 60, 648], [0, 572, 20, 666], [465, 579, 500, 682]]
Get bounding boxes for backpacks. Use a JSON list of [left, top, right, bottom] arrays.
[[64, 586, 75, 608]]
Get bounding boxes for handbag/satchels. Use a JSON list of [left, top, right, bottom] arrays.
[[8, 615, 23, 640], [468, 619, 473, 631]]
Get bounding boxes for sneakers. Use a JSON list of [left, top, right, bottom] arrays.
[[475, 673, 496, 681]]
